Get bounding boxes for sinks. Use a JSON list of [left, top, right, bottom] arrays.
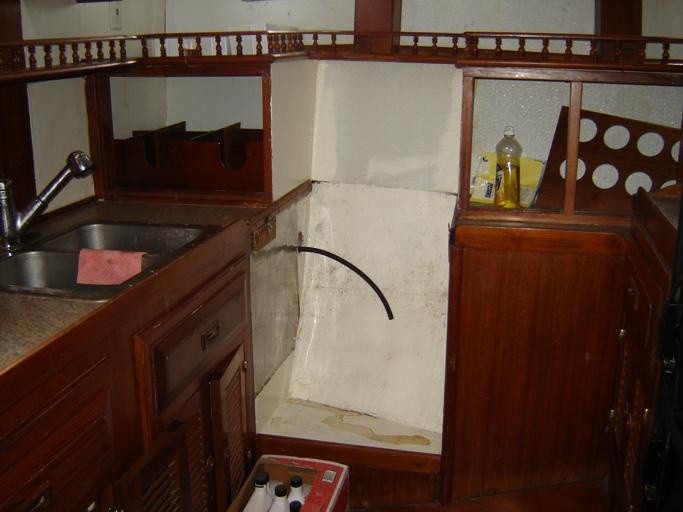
[[38, 220, 222, 253], [0, 249, 166, 304]]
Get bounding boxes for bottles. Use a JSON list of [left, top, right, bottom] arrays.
[[494, 126, 524, 212]]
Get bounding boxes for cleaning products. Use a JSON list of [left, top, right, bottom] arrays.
[[242, 471, 313, 512]]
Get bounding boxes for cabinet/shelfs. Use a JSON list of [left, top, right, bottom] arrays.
[[0, 219, 256, 512], [606, 186, 683, 511], [443, 225, 628, 507]]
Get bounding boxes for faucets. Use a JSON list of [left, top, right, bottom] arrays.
[[0, 151, 94, 250]]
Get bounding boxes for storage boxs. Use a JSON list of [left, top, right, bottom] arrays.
[[225, 454, 351, 512]]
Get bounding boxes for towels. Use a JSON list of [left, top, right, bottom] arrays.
[[77, 248, 147, 286]]
